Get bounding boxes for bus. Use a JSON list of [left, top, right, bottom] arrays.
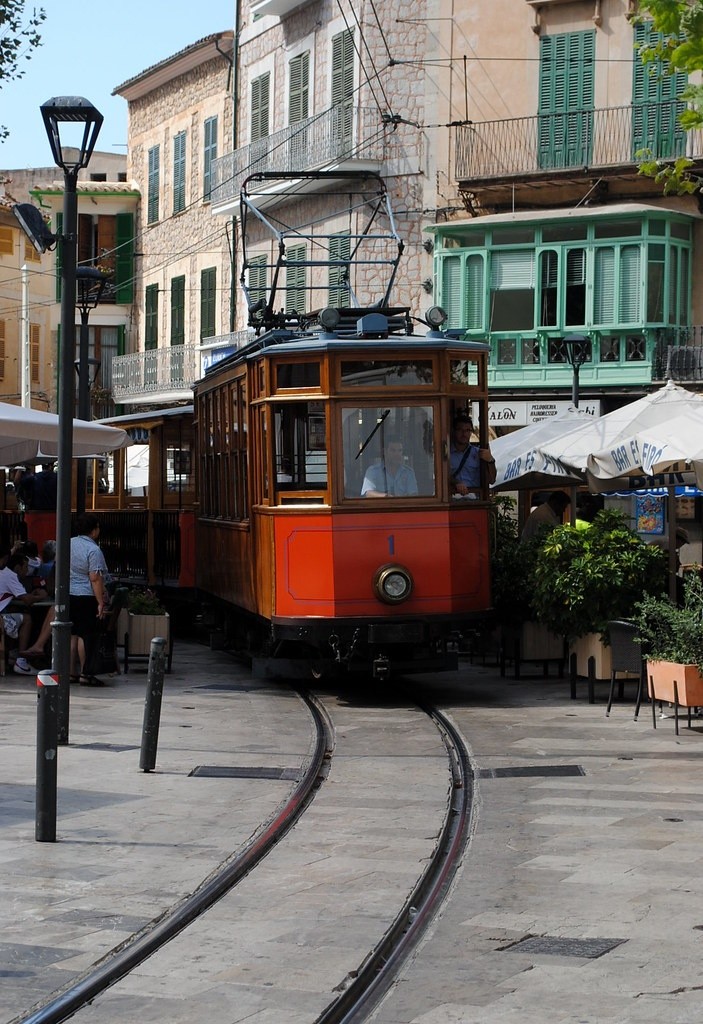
[[67, 168, 501, 706]]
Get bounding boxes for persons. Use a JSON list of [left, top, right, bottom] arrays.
[[0, 538, 55, 654], [359, 437, 419, 498], [568, 506, 595, 529], [87, 460, 109, 493], [0, 554, 41, 675], [446, 414, 497, 495], [517, 491, 572, 553], [19, 465, 58, 510], [68, 517, 109, 687]]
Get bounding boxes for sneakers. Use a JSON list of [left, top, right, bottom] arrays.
[[13, 660, 41, 675]]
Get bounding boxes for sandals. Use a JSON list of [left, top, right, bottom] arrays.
[[70, 674, 79, 683], [78, 674, 105, 687]]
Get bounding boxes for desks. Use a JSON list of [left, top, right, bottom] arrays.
[[9, 602, 66, 670]]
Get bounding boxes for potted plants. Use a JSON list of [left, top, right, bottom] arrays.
[[627, 560, 703, 708], [116, 585, 144, 647], [492, 495, 570, 660], [521, 508, 669, 679], [125, 588, 169, 654]]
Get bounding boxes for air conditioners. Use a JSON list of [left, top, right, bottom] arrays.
[[664, 345, 703, 379]]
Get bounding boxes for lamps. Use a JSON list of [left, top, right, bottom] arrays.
[[11, 203, 63, 253]]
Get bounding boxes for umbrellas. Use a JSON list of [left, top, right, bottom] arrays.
[[487, 405, 601, 529], [537, 380, 703, 603], [0, 401, 134, 466]]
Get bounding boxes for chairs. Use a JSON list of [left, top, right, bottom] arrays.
[[604, 620, 663, 722]]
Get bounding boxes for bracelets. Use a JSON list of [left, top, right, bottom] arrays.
[[106, 485, 109, 486], [488, 459, 495, 464]]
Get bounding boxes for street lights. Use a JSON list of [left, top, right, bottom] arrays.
[[71, 266, 109, 531], [38, 92, 106, 754], [565, 333, 588, 411]]
